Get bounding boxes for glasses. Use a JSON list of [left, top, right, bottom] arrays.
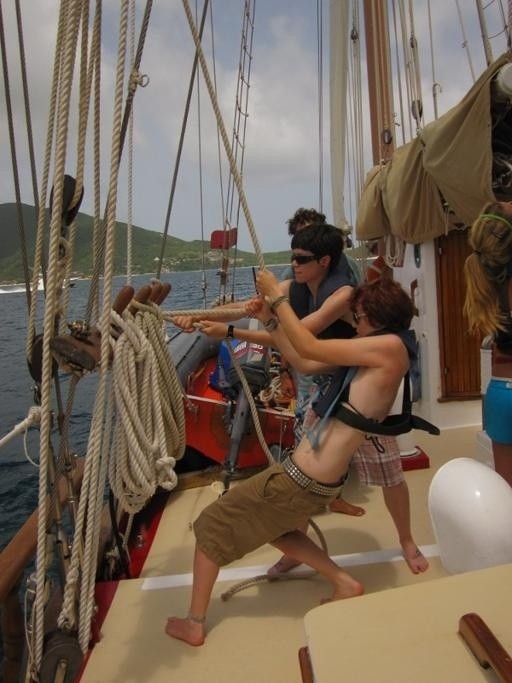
[[291, 256, 316, 265], [353, 313, 366, 324]]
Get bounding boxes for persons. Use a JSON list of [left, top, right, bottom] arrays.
[[169, 221, 429, 581], [468, 199, 512, 488], [278, 206, 366, 286], [164, 271, 414, 648]]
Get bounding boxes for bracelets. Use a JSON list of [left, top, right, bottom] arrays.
[[224, 323, 234, 341], [269, 294, 289, 314], [262, 318, 279, 334]]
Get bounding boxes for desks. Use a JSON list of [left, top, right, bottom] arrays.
[[299, 563, 512, 683]]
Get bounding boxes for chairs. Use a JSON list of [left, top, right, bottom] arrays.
[[427, 457, 512, 573]]
